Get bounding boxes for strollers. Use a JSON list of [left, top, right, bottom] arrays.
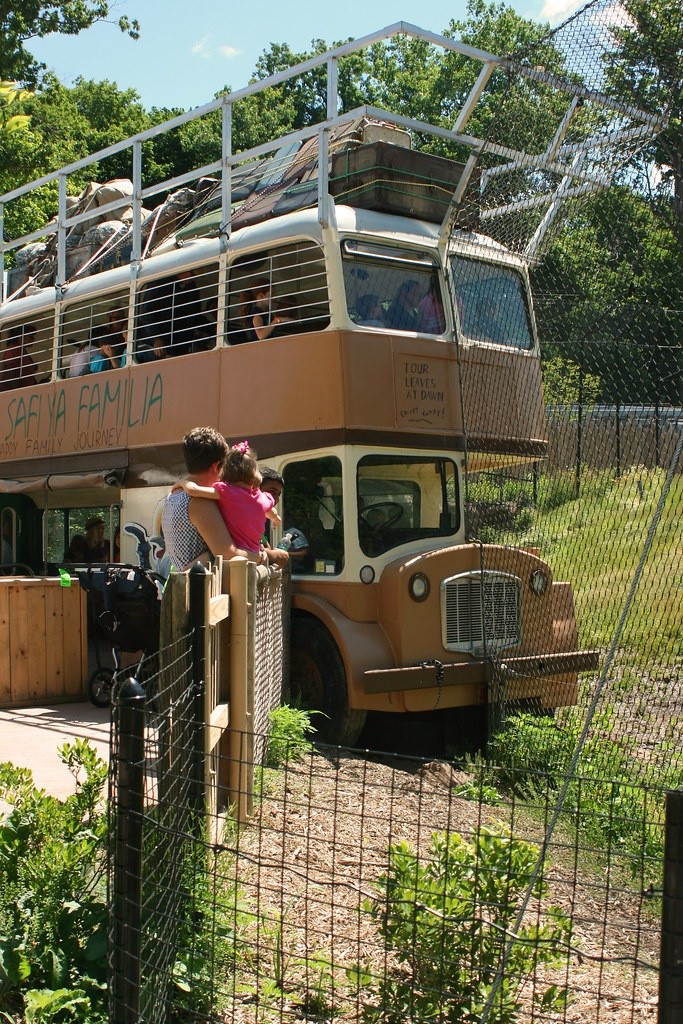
[[80, 562, 162, 708]]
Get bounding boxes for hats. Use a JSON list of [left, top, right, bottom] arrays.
[[85, 518, 106, 530]]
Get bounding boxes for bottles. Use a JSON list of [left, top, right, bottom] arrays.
[[277, 533, 292, 552]]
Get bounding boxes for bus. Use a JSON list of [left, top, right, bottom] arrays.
[[0, 20, 669, 757]]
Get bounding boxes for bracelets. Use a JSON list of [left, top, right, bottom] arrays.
[[274, 317, 281, 323]]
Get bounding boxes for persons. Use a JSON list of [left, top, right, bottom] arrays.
[[331, 497, 371, 539], [0, 325, 38, 392], [68, 516, 120, 563], [195, 296, 218, 326], [238, 277, 299, 339], [469, 294, 506, 343], [418, 274, 467, 335], [160, 426, 290, 574], [355, 294, 389, 328], [140, 272, 217, 359], [283, 457, 342, 575], [385, 279, 427, 332], [62, 305, 128, 378], [273, 294, 298, 318]]
